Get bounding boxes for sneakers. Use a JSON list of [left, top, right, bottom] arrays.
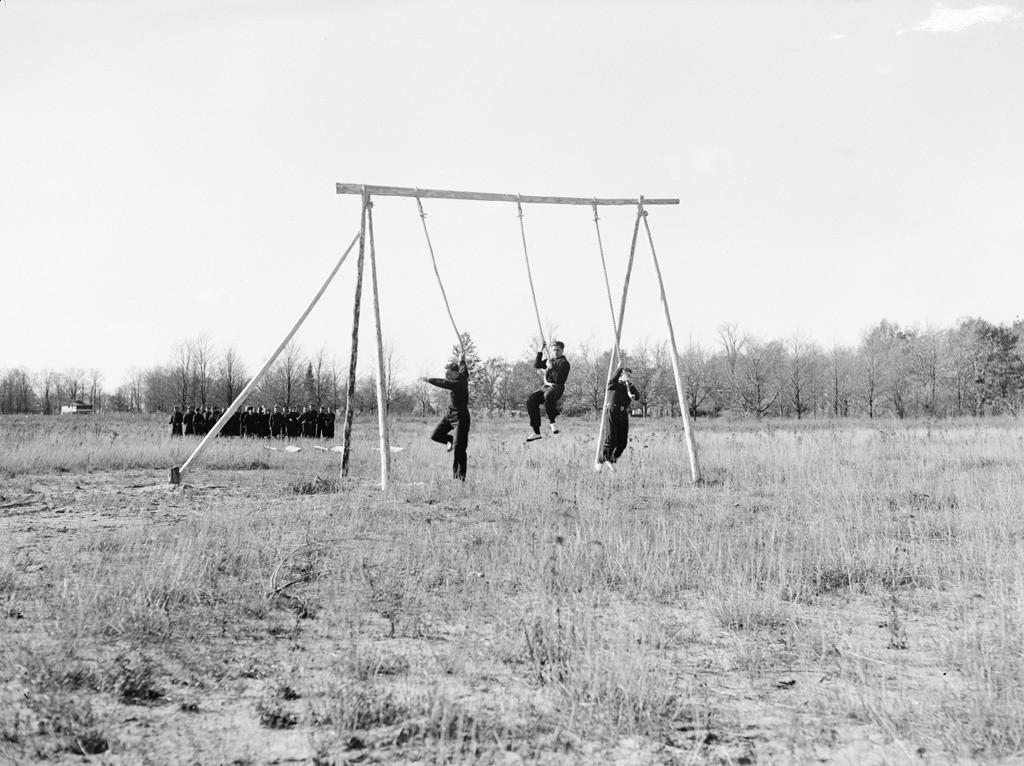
[[550, 422, 559, 434], [527, 433, 542, 442]]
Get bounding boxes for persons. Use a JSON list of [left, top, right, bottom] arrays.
[[169, 404, 335, 439], [595, 362, 640, 474], [419, 350, 472, 482], [526, 340, 571, 441]]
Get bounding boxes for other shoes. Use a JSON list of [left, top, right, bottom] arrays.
[[606, 461, 617, 472], [594, 457, 602, 472], [446, 435, 453, 452]]
